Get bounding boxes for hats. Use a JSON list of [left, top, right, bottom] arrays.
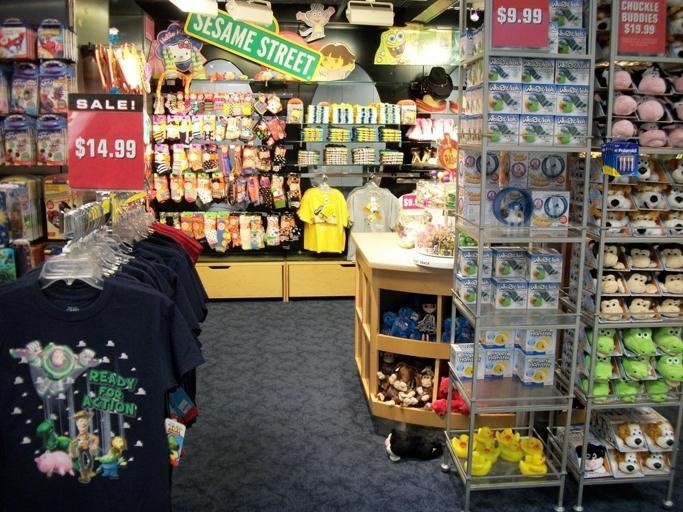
[[415, 67, 467, 115]]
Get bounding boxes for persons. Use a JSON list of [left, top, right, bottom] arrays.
[[384, 429, 443, 463]]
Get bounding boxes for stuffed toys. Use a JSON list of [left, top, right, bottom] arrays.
[[376, 300, 476, 418], [612, 68, 683, 148], [615, 421, 674, 473], [576, 444, 607, 473], [582, 326, 683, 402], [592, 155, 682, 236], [577, 0, 683, 477], [588, 241, 682, 321]]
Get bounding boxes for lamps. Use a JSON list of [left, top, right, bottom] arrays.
[[225, 0, 274, 25]]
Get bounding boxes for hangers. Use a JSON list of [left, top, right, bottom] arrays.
[[366, 172, 379, 190], [38, 190, 161, 290], [320, 174, 331, 191]]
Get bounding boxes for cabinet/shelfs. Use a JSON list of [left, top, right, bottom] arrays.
[[283, 122, 445, 171], [350, 231, 528, 432], [193, 262, 283, 299], [440, 1, 680, 512]]
